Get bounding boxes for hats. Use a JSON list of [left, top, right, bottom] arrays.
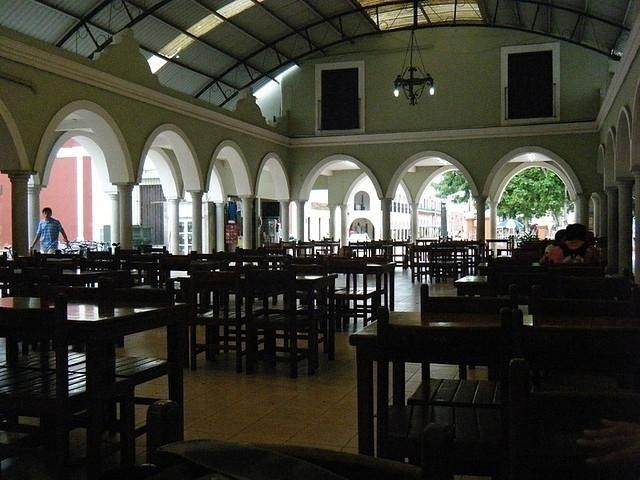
[[564, 223, 586, 242]]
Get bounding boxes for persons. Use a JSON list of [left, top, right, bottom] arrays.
[[30, 207, 70, 255], [576, 417, 640, 468], [546, 224, 597, 264]]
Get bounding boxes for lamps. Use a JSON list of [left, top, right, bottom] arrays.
[[394, 1, 435, 106]]
